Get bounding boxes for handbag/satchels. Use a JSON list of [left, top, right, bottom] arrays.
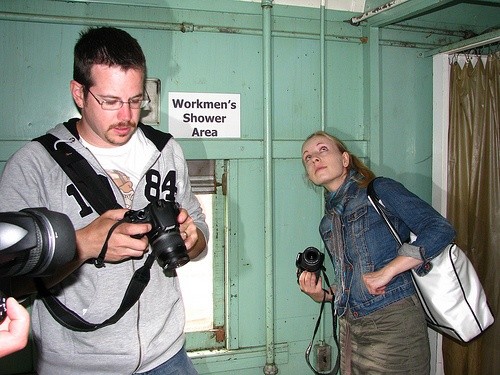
[[409, 232, 495, 346]]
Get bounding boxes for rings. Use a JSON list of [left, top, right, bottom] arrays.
[[182, 231, 188, 239]]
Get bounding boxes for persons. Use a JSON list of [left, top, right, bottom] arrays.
[[299, 131, 456, 375], [0, 296, 31, 358], [0, 26, 210, 375]]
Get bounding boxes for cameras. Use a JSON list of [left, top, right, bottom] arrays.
[[0, 207, 76, 324], [119, 199, 191, 271], [295, 246, 325, 285]]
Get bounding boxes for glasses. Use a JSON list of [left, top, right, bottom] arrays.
[[85, 86, 151, 110]]
